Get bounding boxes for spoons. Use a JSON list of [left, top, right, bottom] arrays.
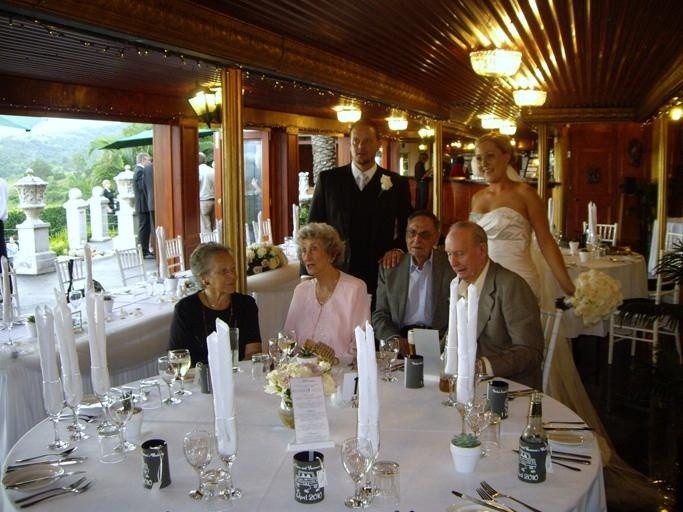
[[13, 445, 80, 463]]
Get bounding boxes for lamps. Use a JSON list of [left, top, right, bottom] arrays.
[[339, 104, 524, 155], [191, 70, 233, 132], [466, 45, 521, 80], [509, 87, 548, 108]]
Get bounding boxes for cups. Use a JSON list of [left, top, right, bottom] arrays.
[[226, 327, 239, 374], [517, 436, 548, 484], [138, 378, 162, 409], [138, 438, 171, 490], [402, 354, 425, 389], [199, 364, 212, 393], [472, 371, 489, 409], [478, 415, 502, 452], [371, 460, 404, 510], [439, 355, 454, 392], [485, 379, 511, 420], [591, 250, 599, 262], [250, 352, 270, 383], [292, 450, 326, 504], [568, 241, 579, 256], [95, 421, 126, 465], [199, 471, 231, 512]]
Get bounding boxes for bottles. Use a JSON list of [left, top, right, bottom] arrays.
[[193, 362, 202, 386], [520, 391, 548, 444]]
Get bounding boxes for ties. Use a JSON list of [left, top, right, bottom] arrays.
[[358, 173, 367, 190]]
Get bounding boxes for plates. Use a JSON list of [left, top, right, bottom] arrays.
[[1, 463, 63, 492], [538, 423, 594, 448], [445, 499, 514, 512]]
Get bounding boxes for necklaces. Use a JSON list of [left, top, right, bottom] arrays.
[[314, 276, 339, 305], [202, 290, 233, 307]]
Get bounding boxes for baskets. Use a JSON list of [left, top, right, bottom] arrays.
[[302, 339, 336, 367]]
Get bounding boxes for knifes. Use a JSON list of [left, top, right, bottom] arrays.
[[451, 487, 508, 512], [507, 446, 595, 475], [6, 455, 91, 471], [541, 419, 596, 432], [5, 469, 86, 489]]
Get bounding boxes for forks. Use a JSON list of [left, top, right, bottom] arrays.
[[13, 477, 93, 510], [471, 480, 541, 512]]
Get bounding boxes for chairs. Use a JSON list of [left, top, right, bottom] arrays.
[[552, 198, 681, 371], [534, 302, 567, 396]]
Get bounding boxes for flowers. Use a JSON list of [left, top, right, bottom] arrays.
[[379, 175, 392, 193]]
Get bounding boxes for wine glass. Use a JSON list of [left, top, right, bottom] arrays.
[[441, 346, 459, 408], [552, 230, 562, 248], [67, 290, 83, 312], [181, 430, 214, 502], [106, 387, 136, 453], [377, 337, 399, 386], [41, 364, 108, 452], [158, 348, 193, 406], [585, 230, 600, 251], [339, 436, 373, 510], [463, 400, 487, 440], [0, 302, 19, 347], [355, 423, 381, 465], [212, 416, 244, 501], [266, 329, 297, 366], [281, 236, 294, 256]]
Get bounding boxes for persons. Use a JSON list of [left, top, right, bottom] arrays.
[[280, 221, 373, 369], [433, 219, 547, 384], [99, 178, 118, 224], [0, 176, 20, 307], [465, 130, 584, 383], [130, 149, 265, 262], [448, 153, 471, 180], [297, 118, 413, 352], [164, 237, 264, 367], [371, 209, 463, 359], [410, 148, 436, 209]]
